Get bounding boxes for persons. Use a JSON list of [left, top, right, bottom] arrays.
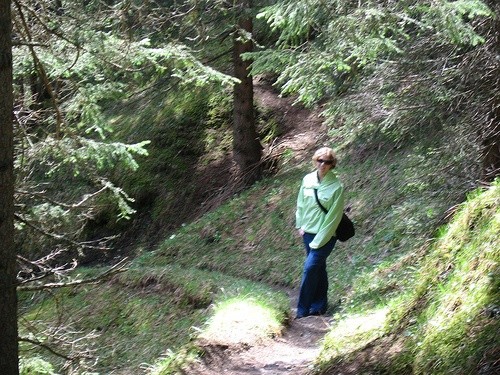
[[294, 147, 343, 320]]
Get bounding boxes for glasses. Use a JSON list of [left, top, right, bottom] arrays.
[[317, 159, 333, 165]]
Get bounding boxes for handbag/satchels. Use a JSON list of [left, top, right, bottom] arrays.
[[335, 213, 355, 242]]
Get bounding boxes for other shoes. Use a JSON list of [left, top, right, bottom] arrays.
[[293, 312, 326, 320]]
